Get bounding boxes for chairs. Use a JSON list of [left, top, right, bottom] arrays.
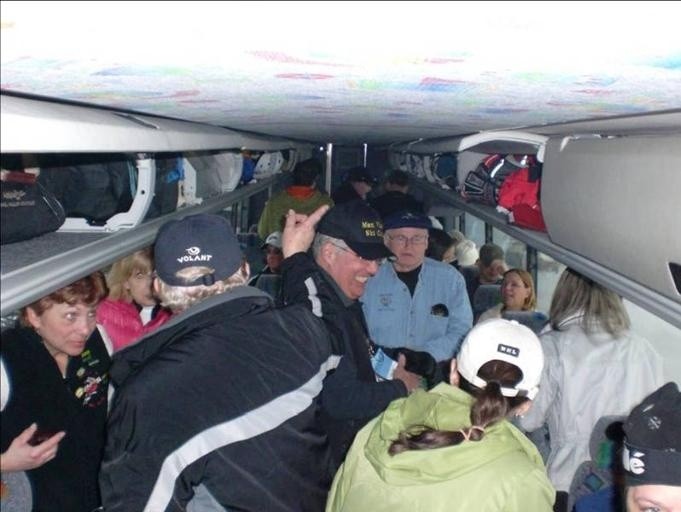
[[457, 264, 550, 335], [234, 223, 285, 311]]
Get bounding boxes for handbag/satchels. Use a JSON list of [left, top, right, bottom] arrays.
[[460, 150, 538, 210], [147, 145, 292, 218], [35, 153, 140, 227], [0, 162, 67, 247], [397, 149, 458, 194], [496, 163, 547, 234]]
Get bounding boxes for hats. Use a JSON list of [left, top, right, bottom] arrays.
[[312, 199, 399, 265], [348, 164, 378, 188], [454, 239, 481, 267], [293, 156, 324, 184], [454, 317, 547, 401], [151, 211, 247, 288], [265, 230, 285, 250], [382, 200, 434, 231]]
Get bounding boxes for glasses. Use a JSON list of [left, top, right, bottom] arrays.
[[264, 247, 282, 256], [387, 234, 429, 244], [328, 241, 389, 267]]
